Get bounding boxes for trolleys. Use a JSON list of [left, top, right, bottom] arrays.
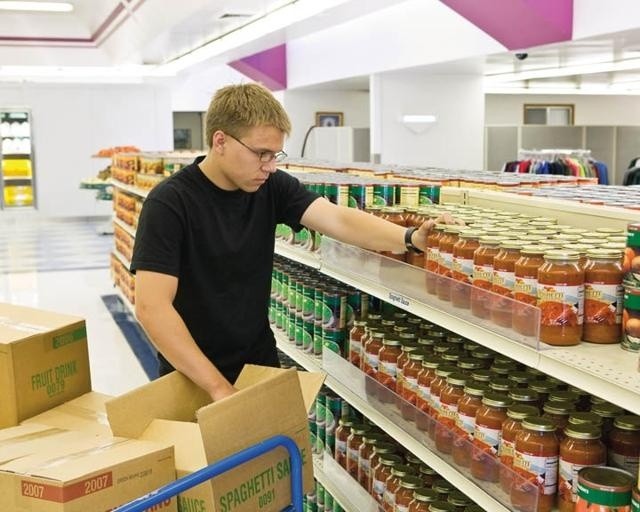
[[98, 433, 315, 512]]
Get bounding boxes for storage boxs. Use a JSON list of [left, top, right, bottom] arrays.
[[20, 391, 118, 435], [105, 363, 328, 511], [0, 301, 92, 431], [1, 422, 177, 512]]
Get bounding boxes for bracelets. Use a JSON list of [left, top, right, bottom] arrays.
[[405, 225, 422, 259]]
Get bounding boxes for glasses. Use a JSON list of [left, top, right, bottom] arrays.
[[223, 132, 287, 162]]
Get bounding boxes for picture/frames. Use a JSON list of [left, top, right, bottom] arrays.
[[316, 111, 343, 127]]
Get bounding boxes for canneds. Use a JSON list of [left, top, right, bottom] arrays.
[[267, 252, 384, 359], [278, 156, 599, 187], [620, 222, 640, 354], [362, 201, 627, 346], [500, 185, 640, 211], [350, 307, 640, 512], [276, 348, 487, 511], [111, 149, 206, 306], [276, 168, 441, 250]]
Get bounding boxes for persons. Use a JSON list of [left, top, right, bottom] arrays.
[[129, 83, 466, 402]]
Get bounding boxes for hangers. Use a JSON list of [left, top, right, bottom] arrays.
[[515, 149, 598, 163]]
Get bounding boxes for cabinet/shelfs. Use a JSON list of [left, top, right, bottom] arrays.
[[112, 151, 640, 510]]
